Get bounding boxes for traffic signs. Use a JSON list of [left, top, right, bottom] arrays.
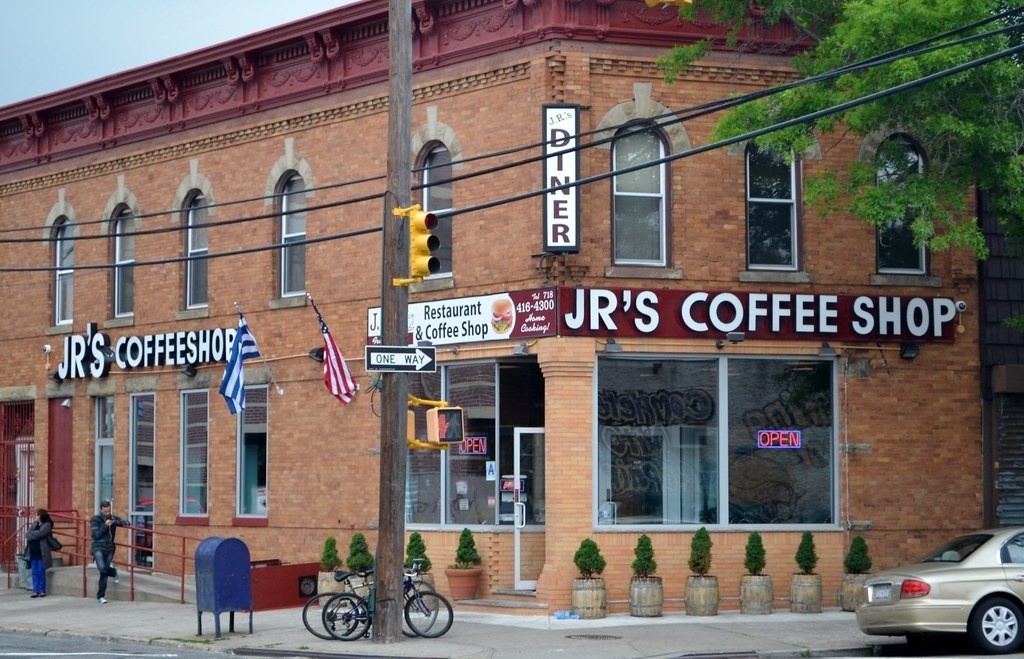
[[365, 344, 437, 373]]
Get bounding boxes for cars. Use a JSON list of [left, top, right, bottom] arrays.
[[855, 526, 1024, 654], [138, 495, 206, 554]]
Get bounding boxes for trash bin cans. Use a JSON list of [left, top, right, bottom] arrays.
[[194, 536, 253, 638]]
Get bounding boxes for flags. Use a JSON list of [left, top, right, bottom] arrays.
[[219, 314, 261, 414], [311, 299, 357, 406]]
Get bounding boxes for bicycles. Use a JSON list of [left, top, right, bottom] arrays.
[[303, 566, 454, 642]]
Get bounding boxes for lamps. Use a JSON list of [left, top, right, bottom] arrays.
[[513, 342, 530, 356], [179, 362, 198, 378], [818, 342, 837, 357], [717, 331, 747, 349], [901, 341, 920, 358], [308, 347, 325, 362], [49, 370, 64, 384], [603, 336, 624, 353], [60, 398, 71, 410]]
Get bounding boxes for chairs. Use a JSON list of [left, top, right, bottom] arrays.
[[942, 551, 960, 561]]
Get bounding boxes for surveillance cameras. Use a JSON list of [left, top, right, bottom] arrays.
[[43, 345, 51, 353], [955, 301, 966, 312]]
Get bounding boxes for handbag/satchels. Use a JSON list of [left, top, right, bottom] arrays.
[[47, 536, 62, 551]]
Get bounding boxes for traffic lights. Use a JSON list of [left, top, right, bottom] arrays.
[[410, 211, 441, 279], [425, 407, 466, 444]]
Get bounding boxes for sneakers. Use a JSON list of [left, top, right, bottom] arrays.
[[113, 568, 120, 583], [98, 597, 107, 604]]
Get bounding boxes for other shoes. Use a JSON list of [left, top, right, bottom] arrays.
[[31, 592, 38, 597], [40, 592, 46, 596]]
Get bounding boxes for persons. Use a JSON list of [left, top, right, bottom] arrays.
[[26, 509, 54, 598], [91, 501, 130, 604]]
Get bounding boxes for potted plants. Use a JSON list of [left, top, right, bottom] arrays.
[[840, 535, 874, 611], [629, 534, 663, 616], [445, 528, 484, 599], [570, 538, 607, 618], [402, 531, 438, 613], [316, 536, 348, 605], [788, 532, 822, 612], [737, 530, 775, 614], [345, 532, 374, 609], [685, 527, 721, 615]]
